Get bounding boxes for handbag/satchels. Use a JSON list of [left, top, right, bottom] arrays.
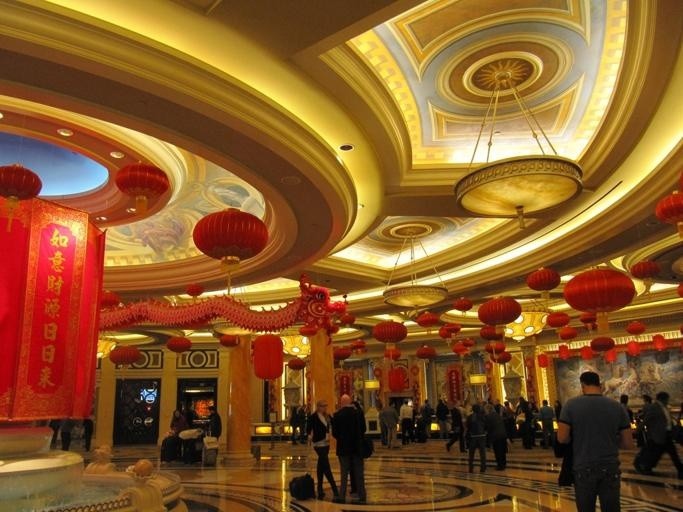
[[349, 436, 376, 459]]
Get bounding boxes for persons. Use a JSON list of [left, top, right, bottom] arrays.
[[60, 418, 78, 451], [82, 419, 93, 453], [182, 401, 195, 426], [170, 410, 190, 458], [49, 418, 60, 448], [205, 406, 222, 458]]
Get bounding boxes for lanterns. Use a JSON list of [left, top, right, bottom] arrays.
[[115, 160, 169, 218], [192, 207, 269, 296], [0, 163, 42, 232], [656, 186, 683, 239]]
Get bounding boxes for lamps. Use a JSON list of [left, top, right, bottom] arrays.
[[468, 373, 486, 400], [364, 379, 381, 406], [97, 330, 117, 357], [452, 70, 583, 228], [502, 301, 552, 345], [280, 335, 311, 359], [382, 227, 448, 317]]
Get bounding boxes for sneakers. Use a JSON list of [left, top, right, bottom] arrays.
[[332, 494, 347, 503], [445, 442, 507, 475], [352, 495, 367, 505]]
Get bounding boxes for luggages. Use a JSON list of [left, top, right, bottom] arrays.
[[159, 435, 177, 464], [287, 450, 316, 501], [201, 431, 220, 468]]
